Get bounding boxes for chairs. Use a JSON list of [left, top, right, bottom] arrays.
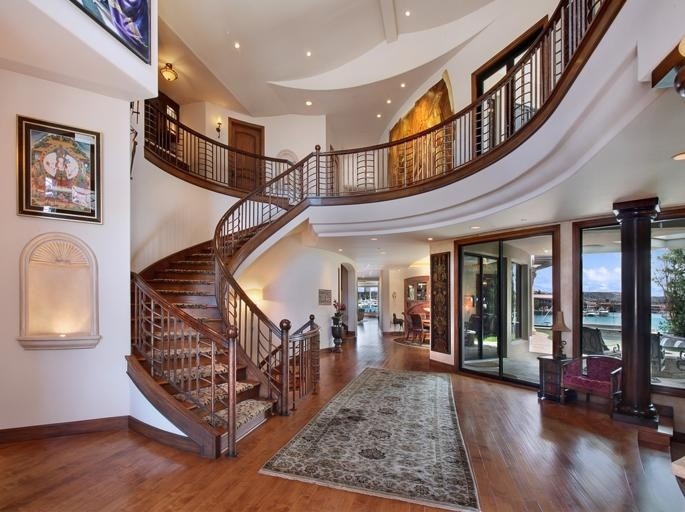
[[561, 355, 622, 420], [402, 312, 413, 340], [409, 313, 430, 344], [581, 327, 621, 359], [651, 332, 666, 377]]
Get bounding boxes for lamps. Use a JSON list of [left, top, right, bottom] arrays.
[[551, 312, 571, 360], [160, 63, 179, 82], [217, 117, 222, 138]]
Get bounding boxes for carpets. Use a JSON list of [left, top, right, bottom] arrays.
[[258, 369, 481, 512], [392, 336, 430, 349]]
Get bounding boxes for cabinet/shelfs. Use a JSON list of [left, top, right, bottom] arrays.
[[476, 273, 497, 336], [403, 276, 430, 335], [537, 356, 573, 404]]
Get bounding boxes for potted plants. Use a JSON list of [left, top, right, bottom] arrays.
[[331, 299, 346, 327], [463, 321, 476, 347]]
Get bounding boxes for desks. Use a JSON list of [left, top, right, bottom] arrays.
[[422, 319, 430, 329]]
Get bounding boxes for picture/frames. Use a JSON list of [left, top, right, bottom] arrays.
[[318, 289, 332, 305], [71, 0, 151, 65], [15, 113, 102, 224]]
